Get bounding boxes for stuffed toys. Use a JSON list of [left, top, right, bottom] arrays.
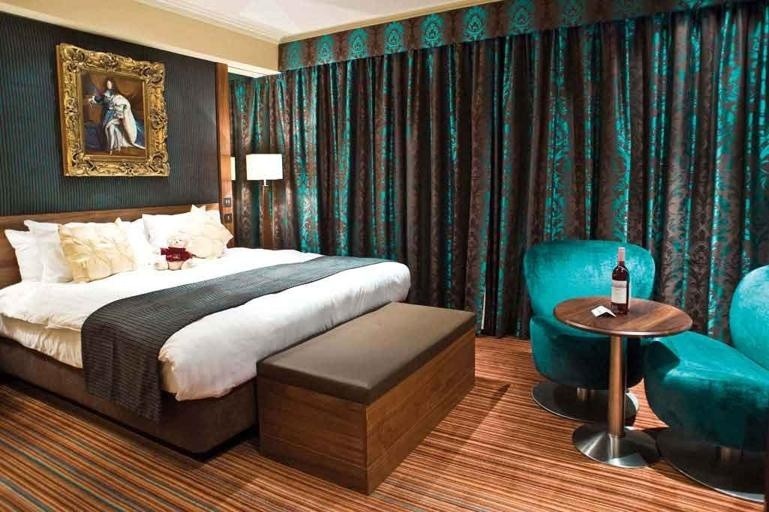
[[154, 231, 198, 271]]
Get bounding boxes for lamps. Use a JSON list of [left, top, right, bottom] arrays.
[[244, 153, 284, 251]]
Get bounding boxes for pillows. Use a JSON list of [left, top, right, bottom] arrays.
[[4, 203, 236, 291]]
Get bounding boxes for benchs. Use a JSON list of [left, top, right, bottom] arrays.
[[256, 299, 477, 494]]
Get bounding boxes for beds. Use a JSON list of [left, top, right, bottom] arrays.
[[0, 198, 412, 463]]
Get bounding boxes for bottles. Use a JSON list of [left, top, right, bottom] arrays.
[[611, 246, 630, 315]]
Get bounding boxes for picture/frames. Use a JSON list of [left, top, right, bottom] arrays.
[[55, 41, 171, 178]]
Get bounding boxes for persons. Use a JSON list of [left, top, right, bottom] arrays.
[[91, 77, 146, 156]]
